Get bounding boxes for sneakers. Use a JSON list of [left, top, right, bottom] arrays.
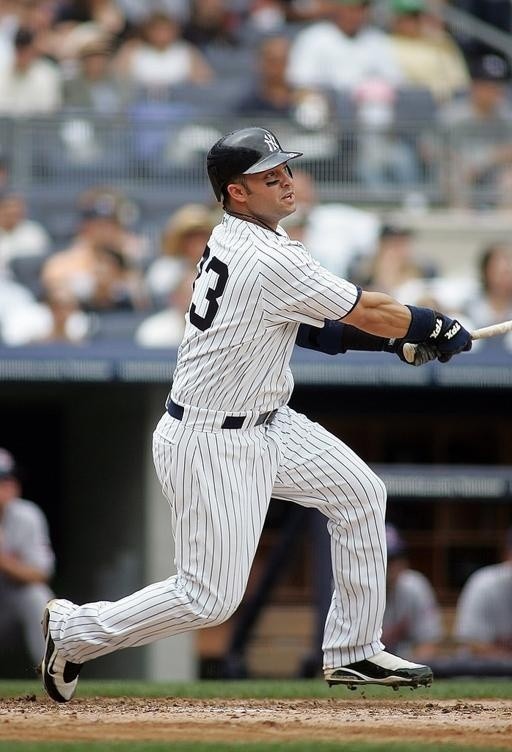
[[324, 651, 433, 691], [41, 599, 81, 703]]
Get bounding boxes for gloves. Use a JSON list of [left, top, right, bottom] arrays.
[[383, 304, 472, 366]]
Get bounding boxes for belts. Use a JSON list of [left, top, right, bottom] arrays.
[[166, 396, 278, 429]]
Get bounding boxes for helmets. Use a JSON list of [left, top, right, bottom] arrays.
[[207, 127, 303, 202]]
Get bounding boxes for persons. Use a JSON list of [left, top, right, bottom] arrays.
[[0, 449, 58, 677], [452, 490, 512, 647], [379, 523, 444, 659], [1, 2, 511, 352], [40, 128, 474, 703]]
